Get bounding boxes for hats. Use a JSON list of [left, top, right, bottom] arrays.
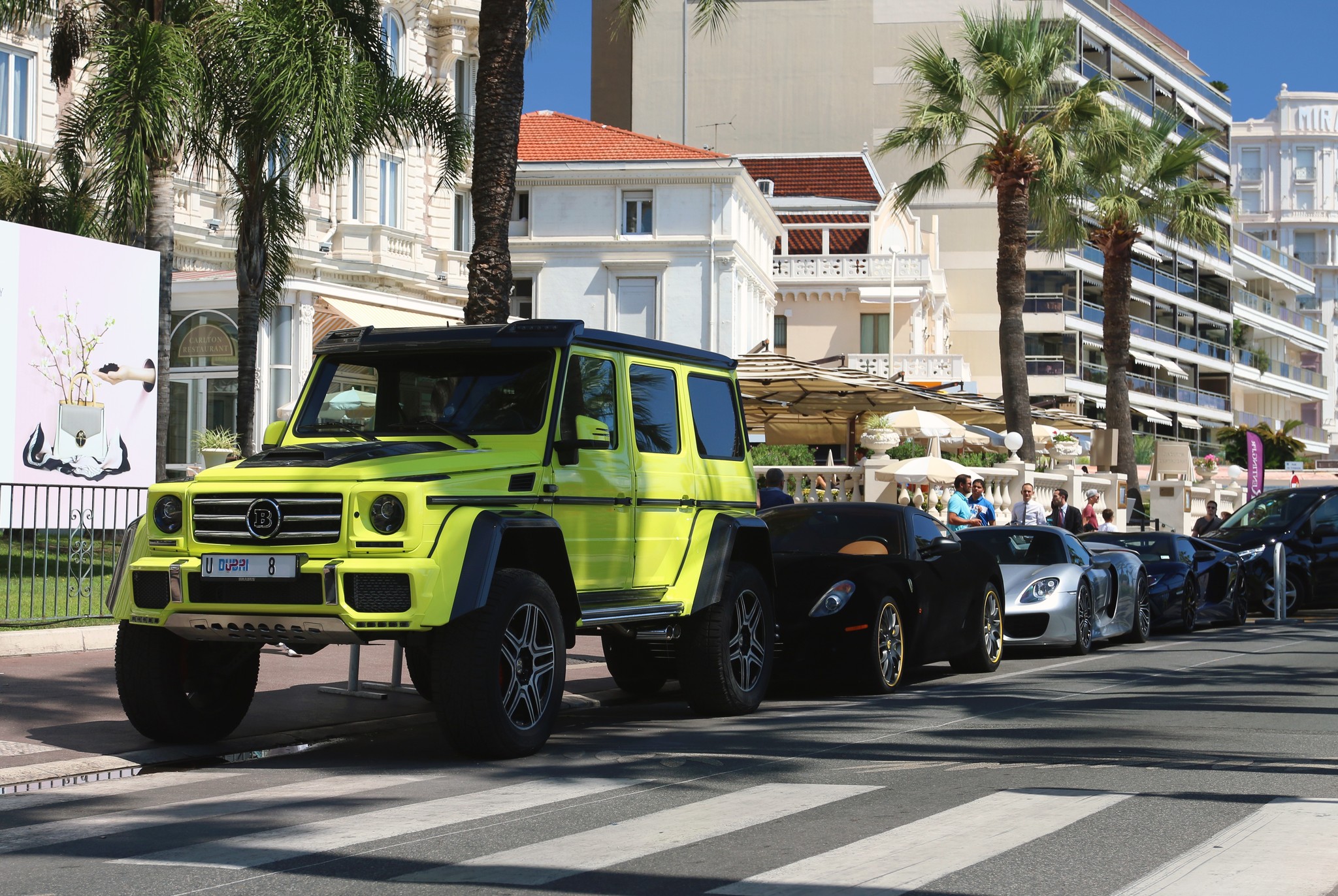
[[1255, 503, 1266, 511], [1085, 489, 1097, 501]]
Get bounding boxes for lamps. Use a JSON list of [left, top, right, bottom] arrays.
[[319, 241, 334, 253], [1005, 432, 1025, 464], [1228, 465, 1244, 488], [437, 271, 448, 283], [204, 218, 223, 232]]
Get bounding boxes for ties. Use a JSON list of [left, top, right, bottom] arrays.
[[1058, 508, 1062, 528], [1022, 503, 1027, 525]]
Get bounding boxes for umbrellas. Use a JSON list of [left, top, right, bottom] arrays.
[[735, 338, 1108, 469], [276, 385, 404, 425]]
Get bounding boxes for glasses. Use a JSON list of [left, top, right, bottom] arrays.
[[1207, 506, 1217, 510], [964, 482, 971, 486], [1094, 495, 1099, 499]]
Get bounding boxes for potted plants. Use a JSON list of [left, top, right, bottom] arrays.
[[191, 426, 243, 471], [859, 407, 901, 460]]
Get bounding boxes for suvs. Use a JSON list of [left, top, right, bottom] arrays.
[[104, 315, 773, 760]]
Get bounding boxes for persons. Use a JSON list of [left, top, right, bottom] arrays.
[[816, 474, 835, 490], [946, 474, 982, 535], [967, 479, 996, 527], [1081, 488, 1099, 532], [1010, 483, 1047, 526], [852, 447, 869, 495], [1046, 489, 1084, 535], [1097, 509, 1118, 532], [92, 364, 156, 385], [1248, 504, 1267, 527], [756, 468, 794, 511], [1190, 501, 1235, 538]]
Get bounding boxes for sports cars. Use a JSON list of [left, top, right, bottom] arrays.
[[747, 501, 1007, 696], [940, 524, 1153, 654], [1075, 530, 1252, 633]]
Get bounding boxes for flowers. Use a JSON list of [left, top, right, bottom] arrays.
[[1051, 430, 1076, 444], [1192, 453, 1223, 472]]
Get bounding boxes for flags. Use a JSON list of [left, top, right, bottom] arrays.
[[1246, 430, 1264, 502]]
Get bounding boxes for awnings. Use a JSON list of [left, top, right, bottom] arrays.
[[1083, 214, 1247, 287], [1084, 396, 1107, 409], [1130, 405, 1173, 426], [1082, 33, 1224, 133], [1083, 336, 1189, 380], [1177, 414, 1202, 429], [1068, 394, 1085, 405]]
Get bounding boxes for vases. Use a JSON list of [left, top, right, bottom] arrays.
[[1195, 465, 1217, 485], [1048, 441, 1084, 470]]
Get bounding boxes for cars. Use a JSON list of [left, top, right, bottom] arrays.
[[1199, 486, 1338, 614]]
